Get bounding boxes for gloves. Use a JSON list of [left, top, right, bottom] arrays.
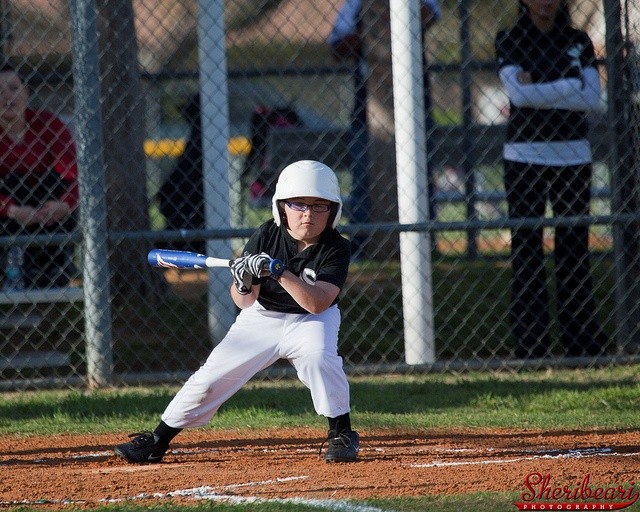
[[244, 252, 279, 280], [230, 251, 252, 295]]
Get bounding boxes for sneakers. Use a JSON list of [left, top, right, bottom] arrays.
[[317, 420, 359, 462], [115, 430, 169, 464]]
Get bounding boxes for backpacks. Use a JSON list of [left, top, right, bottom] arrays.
[[240, 104, 306, 208]]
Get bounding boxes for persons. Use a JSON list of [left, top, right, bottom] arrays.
[[1, 64, 87, 302], [115, 160, 361, 462], [495, 3, 605, 365], [330, 0, 448, 265]]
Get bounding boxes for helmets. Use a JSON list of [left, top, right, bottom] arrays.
[[271, 160, 343, 230]]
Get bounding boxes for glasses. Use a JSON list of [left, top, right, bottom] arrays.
[[284, 200, 332, 213]]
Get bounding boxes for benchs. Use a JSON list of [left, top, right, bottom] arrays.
[[1, 260, 122, 380]]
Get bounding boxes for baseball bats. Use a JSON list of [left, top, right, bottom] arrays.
[[147, 249, 284, 275]]
